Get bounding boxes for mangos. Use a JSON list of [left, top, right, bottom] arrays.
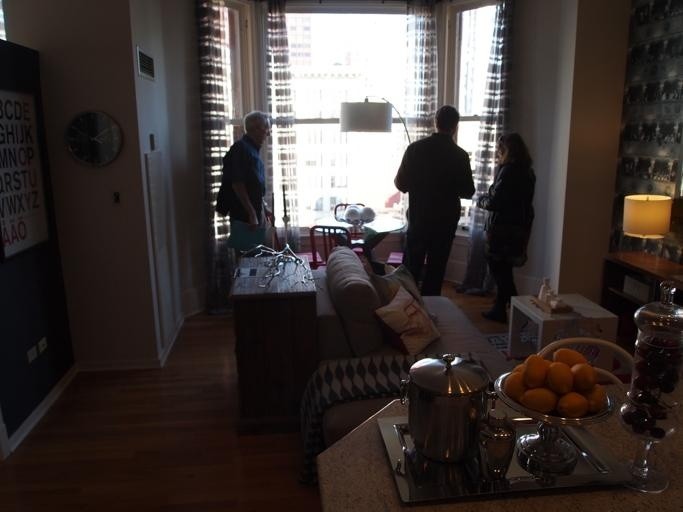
[[504, 347, 609, 417]]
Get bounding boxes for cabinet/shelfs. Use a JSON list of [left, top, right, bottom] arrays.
[[600, 250, 683, 357]]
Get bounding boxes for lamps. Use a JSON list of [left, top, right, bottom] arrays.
[[622, 192, 673, 240]]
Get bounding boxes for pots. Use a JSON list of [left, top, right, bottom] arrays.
[[395, 352, 492, 464]]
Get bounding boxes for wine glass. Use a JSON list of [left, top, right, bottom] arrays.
[[621, 391, 673, 494]]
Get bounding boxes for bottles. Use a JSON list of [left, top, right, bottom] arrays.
[[538, 274, 550, 301], [634, 277, 682, 435]]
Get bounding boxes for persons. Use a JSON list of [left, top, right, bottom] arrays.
[[394, 103, 476, 299], [475, 133, 534, 321], [213, 110, 274, 259], [453, 222, 498, 300]]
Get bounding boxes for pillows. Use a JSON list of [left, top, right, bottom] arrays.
[[363, 263, 437, 322], [373, 285, 441, 354], [324, 245, 385, 359]]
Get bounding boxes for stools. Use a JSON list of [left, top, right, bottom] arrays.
[[295, 251, 324, 267], [387, 252, 428, 267]]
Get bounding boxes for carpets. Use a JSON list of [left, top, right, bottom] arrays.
[[482, 331, 636, 384]]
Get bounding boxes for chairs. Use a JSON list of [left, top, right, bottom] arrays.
[[309, 226, 351, 269]]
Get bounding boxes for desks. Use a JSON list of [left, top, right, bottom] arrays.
[[228, 255, 316, 438]]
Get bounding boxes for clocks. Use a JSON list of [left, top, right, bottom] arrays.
[[64, 111, 123, 167]]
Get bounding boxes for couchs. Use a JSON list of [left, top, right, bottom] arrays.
[[293, 262, 513, 497]]
